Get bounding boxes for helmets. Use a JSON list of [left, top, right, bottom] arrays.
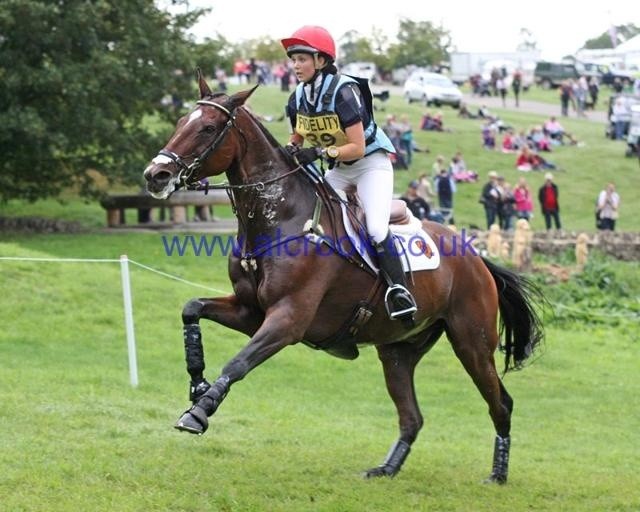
[[281, 26, 336, 63]]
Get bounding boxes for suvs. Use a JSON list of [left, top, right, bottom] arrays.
[[533, 59, 636, 92], [606, 95, 640, 139], [403, 67, 464, 109]]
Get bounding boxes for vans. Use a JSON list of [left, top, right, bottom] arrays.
[[341, 62, 377, 83]]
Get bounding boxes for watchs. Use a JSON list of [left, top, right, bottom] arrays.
[[325, 144, 339, 170]]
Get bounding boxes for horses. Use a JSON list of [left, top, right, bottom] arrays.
[[142, 65, 545, 486]]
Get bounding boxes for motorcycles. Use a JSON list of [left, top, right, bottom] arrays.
[[626, 107, 639, 157]]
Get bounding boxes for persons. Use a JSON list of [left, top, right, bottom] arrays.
[[538, 172, 561, 229], [214, 56, 298, 92], [279, 24, 417, 331], [470, 66, 521, 106], [558, 74, 623, 121], [597, 181, 620, 230], [479, 102, 577, 172], [380, 99, 478, 225], [481, 170, 533, 230]]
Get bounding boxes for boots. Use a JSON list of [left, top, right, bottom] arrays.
[[374, 228, 416, 330]]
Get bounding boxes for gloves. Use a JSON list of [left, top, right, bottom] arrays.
[[294, 147, 317, 168], [285, 146, 297, 155]]
